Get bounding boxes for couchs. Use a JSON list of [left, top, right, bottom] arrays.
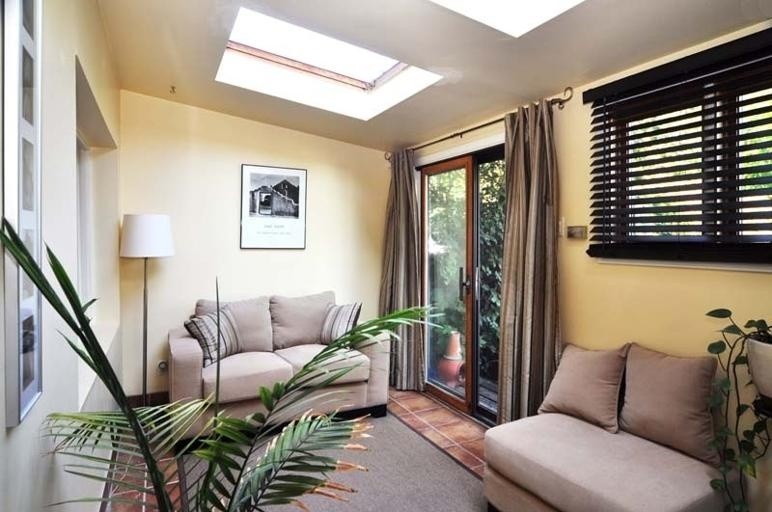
[[166, 320, 391, 457]]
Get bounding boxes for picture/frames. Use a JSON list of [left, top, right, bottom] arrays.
[[240, 164, 307, 250]]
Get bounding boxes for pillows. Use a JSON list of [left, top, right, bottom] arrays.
[[184, 292, 364, 368], [535, 343, 722, 470]]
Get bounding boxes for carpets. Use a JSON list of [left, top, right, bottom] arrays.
[[174, 408, 488, 511]]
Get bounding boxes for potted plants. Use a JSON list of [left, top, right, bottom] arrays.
[[700, 309, 772, 511]]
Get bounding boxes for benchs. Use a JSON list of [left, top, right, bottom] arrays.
[[481, 412, 745, 511]]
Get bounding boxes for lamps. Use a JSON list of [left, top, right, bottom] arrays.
[[118, 212, 174, 407]]
